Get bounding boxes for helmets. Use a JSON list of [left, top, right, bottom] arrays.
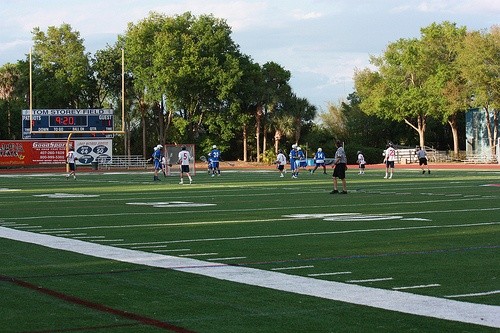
[[181, 145, 186, 150], [292, 145, 297, 150], [297, 146, 301, 151], [318, 148, 322, 152], [212, 145, 216, 148]]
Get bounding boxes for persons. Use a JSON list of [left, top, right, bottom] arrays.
[[384, 143, 396, 179], [206, 145, 220, 177], [152, 145, 164, 182], [276, 144, 306, 179], [329, 140, 348, 194], [311, 148, 326, 175], [416, 147, 431, 174], [66, 146, 78, 181], [178, 146, 193, 185], [357, 151, 366, 175]]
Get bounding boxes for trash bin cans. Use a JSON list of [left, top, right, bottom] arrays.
[[91, 161, 98, 170]]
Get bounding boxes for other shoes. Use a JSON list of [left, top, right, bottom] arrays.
[[359, 173, 361, 175], [329, 189, 338, 194], [324, 172, 327, 174], [362, 173, 365, 175], [280, 173, 299, 179], [340, 190, 347, 194], [428, 170, 431, 174], [312, 170, 314, 173], [423, 170, 425, 174], [309, 169, 312, 172], [66, 175, 69, 180], [389, 177, 392, 179], [74, 176, 78, 180], [208, 172, 221, 177], [384, 176, 388, 179], [190, 179, 193, 184], [179, 181, 183, 184], [154, 179, 161, 182]]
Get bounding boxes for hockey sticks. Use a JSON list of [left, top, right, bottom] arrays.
[[220, 158, 235, 166], [200, 156, 208, 165]]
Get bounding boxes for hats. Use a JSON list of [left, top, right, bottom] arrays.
[[157, 145, 163, 148]]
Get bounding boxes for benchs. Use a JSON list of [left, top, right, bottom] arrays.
[[383, 149, 419, 164], [461, 154, 498, 164], [97, 156, 148, 170]]
